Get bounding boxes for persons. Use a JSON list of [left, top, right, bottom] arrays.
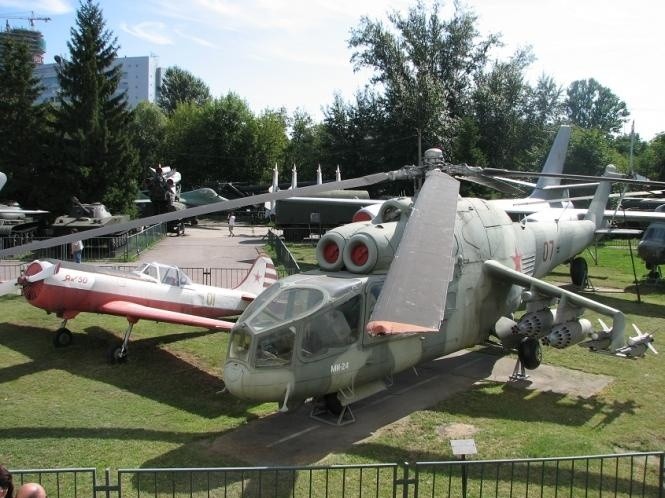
[[0, 466, 14, 498], [14, 482, 47, 498], [70, 229, 84, 263], [227, 211, 236, 237]]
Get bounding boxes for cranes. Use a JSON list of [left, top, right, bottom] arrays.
[[0, 8, 52, 29]]
